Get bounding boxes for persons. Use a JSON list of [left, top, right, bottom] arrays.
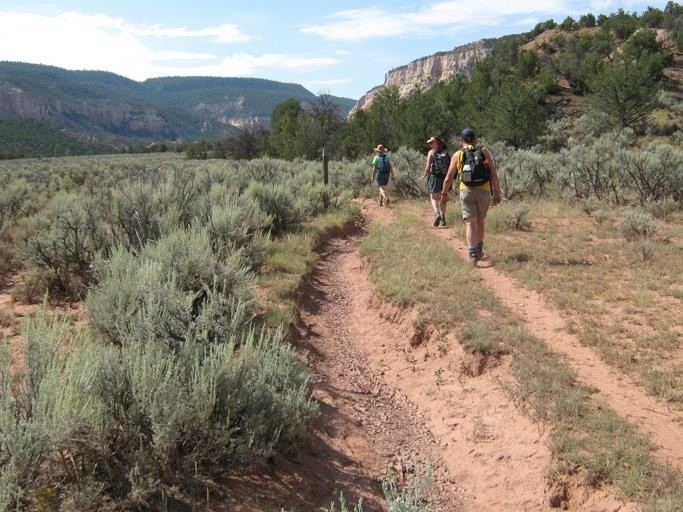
[[372, 144, 395, 207], [421, 136, 453, 226], [441, 128, 502, 264]]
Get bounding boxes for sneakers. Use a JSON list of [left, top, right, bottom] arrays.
[[441, 217, 446, 226], [475, 246, 482, 260], [470, 253, 477, 266], [379, 197, 390, 208], [434, 214, 441, 227]]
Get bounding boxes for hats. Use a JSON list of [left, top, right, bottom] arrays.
[[372, 144, 388, 152], [426, 137, 442, 144], [458, 128, 475, 143]]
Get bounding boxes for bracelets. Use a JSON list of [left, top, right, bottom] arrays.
[[442, 192, 448, 196]]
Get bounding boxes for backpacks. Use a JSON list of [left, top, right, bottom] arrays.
[[377, 154, 390, 173], [460, 146, 490, 187], [430, 151, 451, 178]]
